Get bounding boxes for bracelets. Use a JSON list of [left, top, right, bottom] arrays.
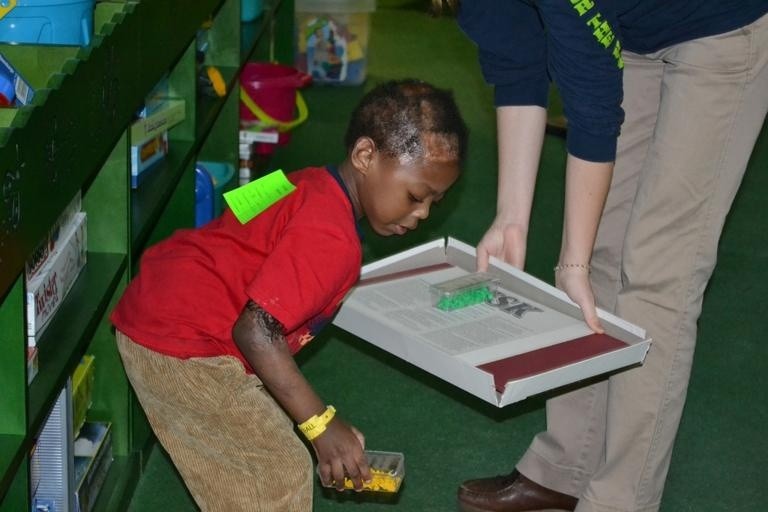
[[552, 264, 592, 273], [296, 404, 337, 441]]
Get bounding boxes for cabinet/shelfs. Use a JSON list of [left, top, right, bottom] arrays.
[[0, 0, 296, 512]]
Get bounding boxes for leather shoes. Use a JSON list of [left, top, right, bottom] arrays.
[[456, 468, 578, 512]]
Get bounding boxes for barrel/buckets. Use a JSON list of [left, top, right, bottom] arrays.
[[239, 60, 315, 156], [0, 0, 96, 44]]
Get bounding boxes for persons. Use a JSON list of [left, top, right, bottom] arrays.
[[425, 0, 765, 510], [109, 74, 469, 511]]
